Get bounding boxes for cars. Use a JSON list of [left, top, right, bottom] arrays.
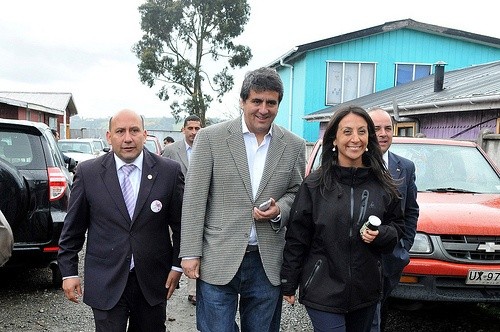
[[305, 136, 500, 304], [144, 134, 164, 157], [58, 137, 110, 167]]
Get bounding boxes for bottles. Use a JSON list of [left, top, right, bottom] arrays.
[[359, 215, 381, 243]]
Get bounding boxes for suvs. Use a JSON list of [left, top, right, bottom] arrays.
[[0, 116, 75, 258]]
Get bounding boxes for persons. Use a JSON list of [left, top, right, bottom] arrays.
[[280, 106, 405, 332], [178, 67, 306, 332], [161, 115, 205, 306], [57, 109, 186, 332], [366, 108, 420, 332], [163, 136, 175, 149]]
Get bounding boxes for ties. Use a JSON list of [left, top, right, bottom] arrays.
[[120, 164, 136, 219]]
